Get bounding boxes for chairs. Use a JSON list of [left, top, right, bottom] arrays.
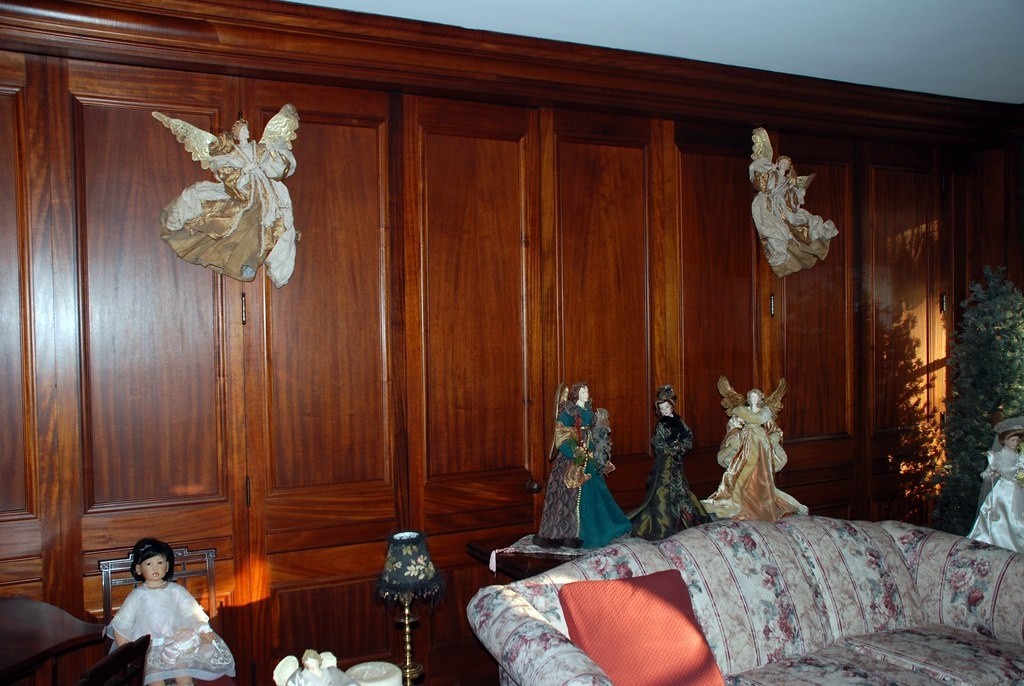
[[78, 634, 151, 686], [99, 547, 236, 686]]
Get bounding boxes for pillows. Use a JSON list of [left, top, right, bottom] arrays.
[[558, 570, 727, 686]]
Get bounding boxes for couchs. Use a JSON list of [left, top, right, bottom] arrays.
[[466, 515, 1024, 686]]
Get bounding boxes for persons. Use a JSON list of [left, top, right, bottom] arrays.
[[747, 123, 838, 276], [533, 377, 634, 549], [107, 536, 236, 686], [149, 102, 300, 286], [630, 387, 714, 540], [700, 372, 810, 519], [967, 425, 1024, 555]]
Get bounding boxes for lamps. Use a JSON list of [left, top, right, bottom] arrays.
[[376, 531, 443, 686]]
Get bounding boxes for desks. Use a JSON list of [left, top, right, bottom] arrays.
[[0, 596, 106, 686]]
